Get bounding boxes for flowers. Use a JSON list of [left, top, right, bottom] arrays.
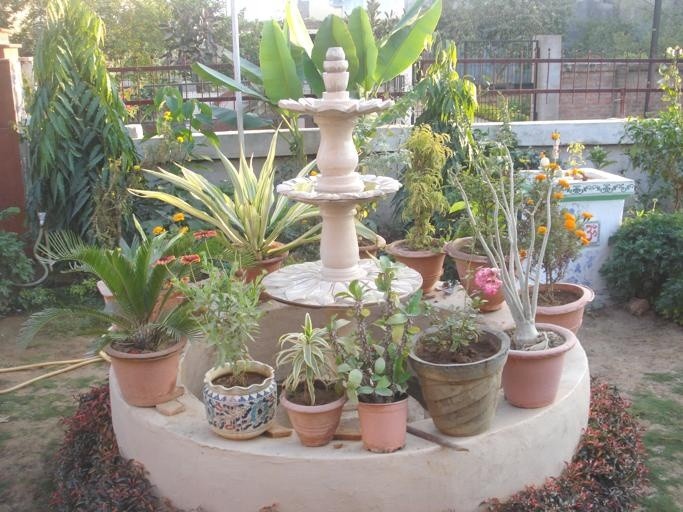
[[518, 130, 596, 299], [458, 266, 502, 347]]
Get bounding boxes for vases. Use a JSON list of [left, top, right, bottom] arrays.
[[273, 312, 350, 448], [327, 259, 423, 452], [525, 282, 595, 337]]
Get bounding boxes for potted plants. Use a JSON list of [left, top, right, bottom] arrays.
[[408, 234, 512, 437], [35, 230, 201, 409], [386, 123, 456, 294], [344, 120, 386, 259], [176, 249, 278, 441], [98, 121, 325, 328], [443, 135, 517, 311], [448, 121, 576, 410]]
